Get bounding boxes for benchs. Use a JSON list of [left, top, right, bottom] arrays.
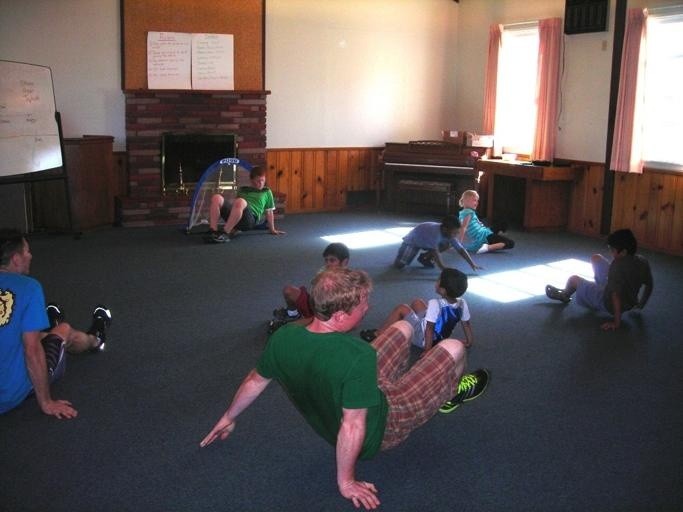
[[398, 180, 454, 219]]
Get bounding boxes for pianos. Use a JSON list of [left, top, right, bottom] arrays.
[[383, 142, 493, 219]]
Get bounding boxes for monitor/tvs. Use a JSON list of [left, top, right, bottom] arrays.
[[160, 131, 236, 192]]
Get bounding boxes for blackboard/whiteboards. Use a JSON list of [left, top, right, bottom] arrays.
[[0, 60, 64, 179]]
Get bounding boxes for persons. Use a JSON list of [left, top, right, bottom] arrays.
[[200, 268, 489, 510], [0, 228, 112, 420], [202, 167, 286, 243], [458, 190, 515, 255], [395, 214, 486, 272], [361, 269, 472, 358], [547, 228, 653, 333], [275, 242, 349, 321]]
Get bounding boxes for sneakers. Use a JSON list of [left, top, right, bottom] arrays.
[[438, 367, 490, 413], [545, 285, 571, 304], [201, 228, 219, 240], [213, 231, 230, 243], [273, 307, 301, 321], [360, 330, 377, 343], [267, 320, 287, 335], [367, 329, 377, 334], [87, 304, 112, 353], [476, 244, 489, 254], [417, 253, 435, 268], [45, 302, 64, 333]]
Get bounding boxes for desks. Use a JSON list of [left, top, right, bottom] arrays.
[[477, 160, 576, 231]]
